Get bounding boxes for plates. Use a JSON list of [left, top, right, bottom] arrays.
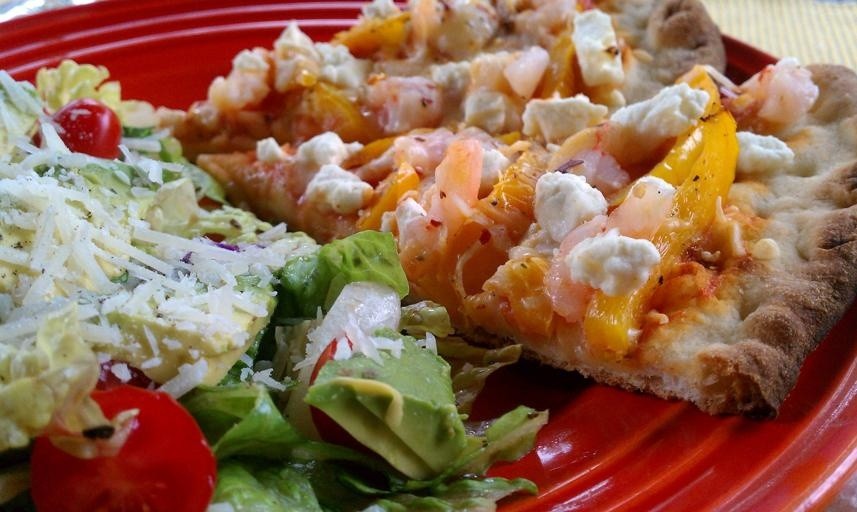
[[0, 0, 857, 512]]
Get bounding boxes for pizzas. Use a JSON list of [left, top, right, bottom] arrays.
[[156, 0, 857, 417]]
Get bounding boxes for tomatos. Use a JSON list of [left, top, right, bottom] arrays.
[[28, 382, 216, 512], [33, 99, 121, 163]]
